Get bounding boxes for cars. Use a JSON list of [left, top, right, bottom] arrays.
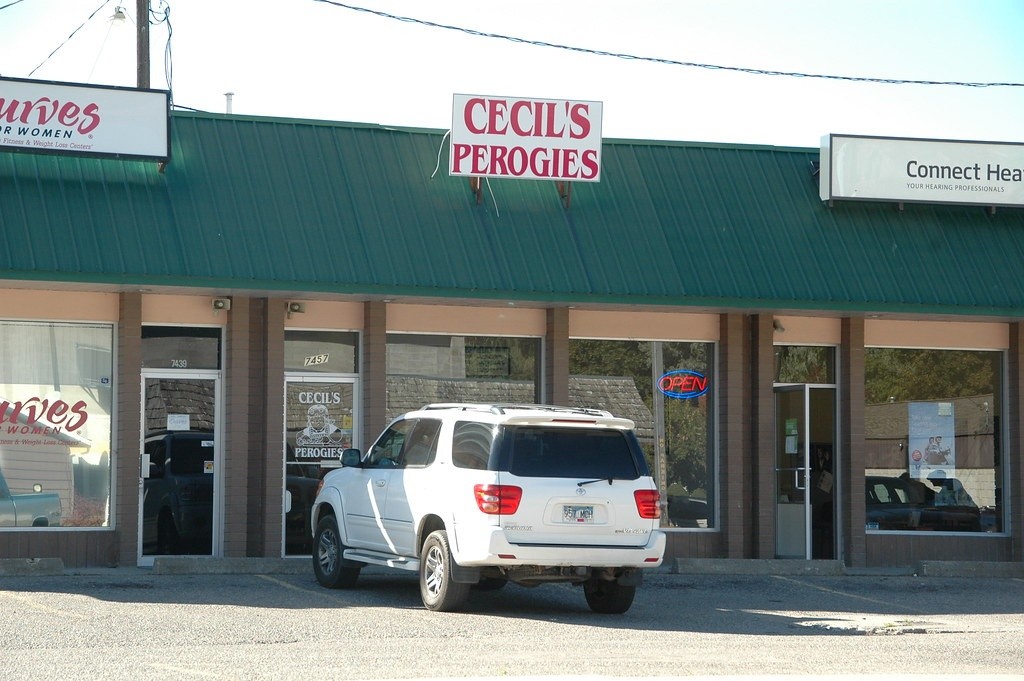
[[865, 475, 981, 532]]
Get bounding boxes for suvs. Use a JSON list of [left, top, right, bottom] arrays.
[[309, 401, 668, 613], [144, 427, 322, 554]]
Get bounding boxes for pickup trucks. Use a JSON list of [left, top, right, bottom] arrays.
[[1, 469, 61, 527]]
